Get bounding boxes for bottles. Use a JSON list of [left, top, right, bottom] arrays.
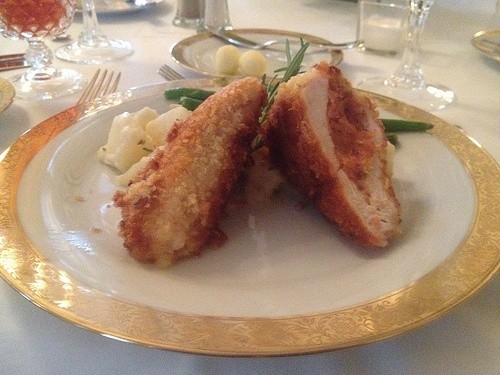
[[171, 0, 229, 28]]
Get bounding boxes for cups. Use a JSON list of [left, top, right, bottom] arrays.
[[356, 0, 411, 56]]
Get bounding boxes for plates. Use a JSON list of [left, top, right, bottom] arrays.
[[0, 78, 500, 358], [76, 0, 165, 15], [170, 27, 344, 81], [471, 27, 500, 61]]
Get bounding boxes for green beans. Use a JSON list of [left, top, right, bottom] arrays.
[[164, 86, 433, 144]]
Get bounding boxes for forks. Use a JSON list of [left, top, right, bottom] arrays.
[[156, 63, 185, 82], [75, 67, 122, 105]]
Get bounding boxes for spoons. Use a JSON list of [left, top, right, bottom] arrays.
[[203, 24, 363, 54]]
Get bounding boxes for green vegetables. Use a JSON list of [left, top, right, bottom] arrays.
[[249, 37, 310, 150]]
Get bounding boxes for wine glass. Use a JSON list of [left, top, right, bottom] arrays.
[[355, 0, 456, 112], [55, 0, 134, 64], [0, 0, 88, 101]]
